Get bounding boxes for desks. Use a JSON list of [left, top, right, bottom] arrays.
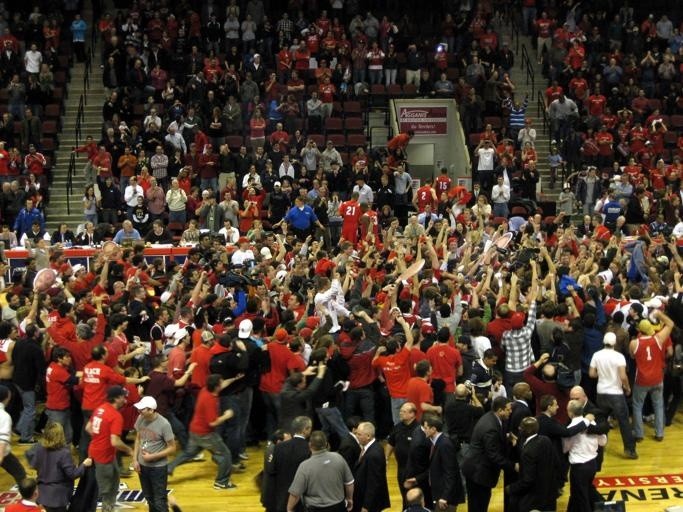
[[3, 245, 254, 285]]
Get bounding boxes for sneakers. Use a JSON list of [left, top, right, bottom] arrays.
[[623, 450, 639, 460], [213, 481, 237, 489], [653, 436, 665, 441], [635, 432, 644, 443], [239, 449, 249, 459], [233, 460, 246, 471]]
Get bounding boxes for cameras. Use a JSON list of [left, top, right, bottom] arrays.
[[463, 379, 472, 389]]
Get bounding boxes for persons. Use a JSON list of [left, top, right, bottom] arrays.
[[2, 1, 69, 244], [1, 219, 682, 511], [71, 2, 542, 245], [508, 3, 682, 236], [70, 15, 88, 63]]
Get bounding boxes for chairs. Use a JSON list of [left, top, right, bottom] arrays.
[[134, 53, 415, 240], [0, 32, 74, 195], [579, 98, 683, 163], [469, 117, 558, 231]]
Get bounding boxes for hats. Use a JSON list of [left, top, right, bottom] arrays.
[[222, 317, 233, 326], [238, 236, 251, 243], [644, 298, 662, 308], [602, 331, 617, 346], [275, 328, 288, 344], [306, 315, 320, 327], [511, 311, 526, 330], [276, 270, 287, 283], [132, 396, 158, 410], [212, 324, 224, 333], [261, 246, 272, 259], [299, 328, 312, 337], [164, 323, 187, 346], [640, 319, 655, 336], [200, 330, 214, 342], [238, 318, 253, 338], [105, 385, 127, 397]]
